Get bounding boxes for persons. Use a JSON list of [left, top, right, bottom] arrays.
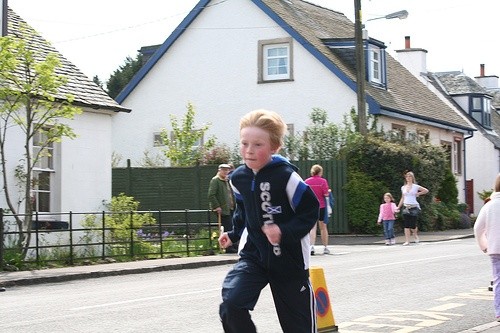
[[473, 173, 500, 322], [377, 193, 400, 245], [218, 110, 321, 333], [208, 164, 238, 253], [303, 164, 331, 255], [397, 171, 429, 246]]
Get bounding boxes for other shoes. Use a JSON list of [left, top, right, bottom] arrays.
[[385, 240, 389, 245], [226, 247, 237, 253], [324, 249, 329, 254], [311, 248, 314, 255], [391, 237, 396, 244], [407, 206, 419, 217], [403, 242, 409, 246]]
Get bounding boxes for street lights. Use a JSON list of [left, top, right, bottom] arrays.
[[355, 8, 409, 133]]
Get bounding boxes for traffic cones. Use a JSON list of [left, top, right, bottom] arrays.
[[307, 266, 342, 333]]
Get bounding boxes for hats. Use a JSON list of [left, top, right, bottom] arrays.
[[218, 164, 230, 169]]
